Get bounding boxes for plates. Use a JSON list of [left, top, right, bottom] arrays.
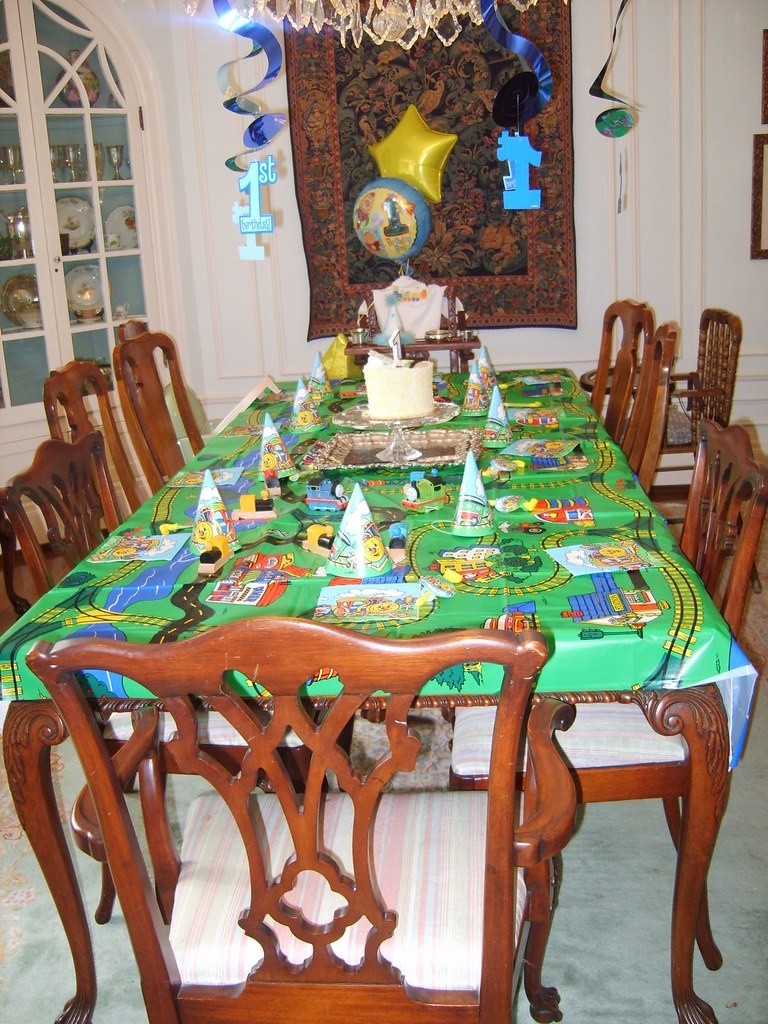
[[106, 206, 137, 249], [65, 264, 112, 317], [0, 274, 41, 326], [57, 195, 95, 250]]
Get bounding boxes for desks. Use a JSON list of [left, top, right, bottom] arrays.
[[0, 370, 759, 1022], [344, 333, 481, 372]]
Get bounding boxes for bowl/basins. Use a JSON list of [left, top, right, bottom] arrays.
[[425, 330, 453, 344]]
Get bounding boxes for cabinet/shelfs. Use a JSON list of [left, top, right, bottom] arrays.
[[0, 0, 168, 445]]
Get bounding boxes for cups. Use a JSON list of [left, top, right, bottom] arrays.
[[0, 237, 12, 259], [460, 330, 472, 343], [75, 308, 95, 319], [351, 328, 371, 346], [59, 233, 69, 255], [105, 233, 120, 251]]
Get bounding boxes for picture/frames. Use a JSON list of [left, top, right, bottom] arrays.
[[748, 134, 766, 260]]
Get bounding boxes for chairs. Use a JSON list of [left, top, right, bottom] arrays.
[[617, 320, 682, 498], [114, 320, 205, 492], [588, 300, 656, 464], [578, 307, 744, 476]]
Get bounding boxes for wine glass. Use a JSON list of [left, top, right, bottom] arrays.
[[63, 143, 83, 182], [0, 144, 21, 185], [107, 143, 124, 180], [48, 142, 59, 182]]
